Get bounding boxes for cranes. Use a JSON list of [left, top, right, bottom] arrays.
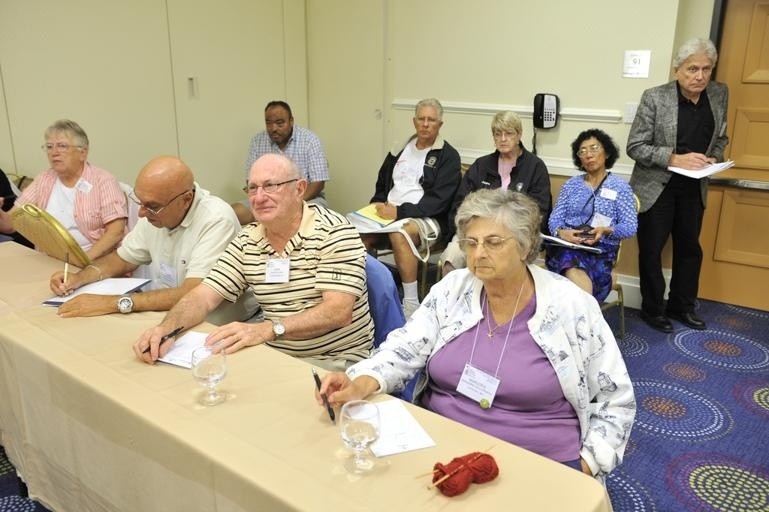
[[540, 234, 602, 255]]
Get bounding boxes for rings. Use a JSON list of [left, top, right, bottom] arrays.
[[686, 148, 713, 166], [384, 200, 389, 206], [312, 366, 335, 422], [142, 325, 185, 354], [61, 252, 70, 298]]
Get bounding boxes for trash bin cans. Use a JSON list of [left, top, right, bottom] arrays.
[[264, 317, 285, 339], [116, 293, 134, 314]]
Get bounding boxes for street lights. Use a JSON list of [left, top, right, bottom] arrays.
[[345, 212, 439, 263]]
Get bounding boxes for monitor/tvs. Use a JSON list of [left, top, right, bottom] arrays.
[[539, 233, 602, 254], [42, 276, 153, 307], [668, 160, 736, 179], [351, 202, 396, 228]]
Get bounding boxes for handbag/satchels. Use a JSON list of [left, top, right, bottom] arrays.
[[86, 264, 104, 283]]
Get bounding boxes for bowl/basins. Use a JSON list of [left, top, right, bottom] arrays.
[[482, 289, 532, 339]]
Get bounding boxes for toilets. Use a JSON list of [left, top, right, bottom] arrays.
[[531, 93, 561, 132]]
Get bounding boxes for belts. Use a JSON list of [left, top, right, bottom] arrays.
[[190, 344, 229, 408], [339, 399, 381, 473]]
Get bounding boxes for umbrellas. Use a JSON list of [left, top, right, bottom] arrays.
[[639, 307, 674, 333], [666, 311, 706, 329]]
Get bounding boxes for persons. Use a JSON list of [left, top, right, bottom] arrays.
[[45, 153, 265, 328], [0, 118, 128, 266], [543, 129, 638, 305], [224, 100, 329, 227], [132, 152, 375, 378], [312, 185, 636, 485], [625, 35, 731, 336], [448, 110, 554, 238], [355, 95, 462, 323]]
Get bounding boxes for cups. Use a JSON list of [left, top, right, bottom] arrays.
[[127, 189, 190, 216], [576, 143, 602, 158], [242, 179, 298, 193], [456, 236, 514, 253], [41, 142, 83, 153]]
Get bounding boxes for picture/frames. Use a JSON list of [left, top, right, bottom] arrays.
[[1, 239, 617, 512]]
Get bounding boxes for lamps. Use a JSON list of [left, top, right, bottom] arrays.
[[115, 181, 143, 237], [364, 252, 408, 350], [5, 171, 35, 195], [9, 200, 94, 271], [577, 191, 641, 343], [371, 165, 473, 300]]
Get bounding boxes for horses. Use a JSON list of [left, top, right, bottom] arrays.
[[576, 233, 594, 238]]
